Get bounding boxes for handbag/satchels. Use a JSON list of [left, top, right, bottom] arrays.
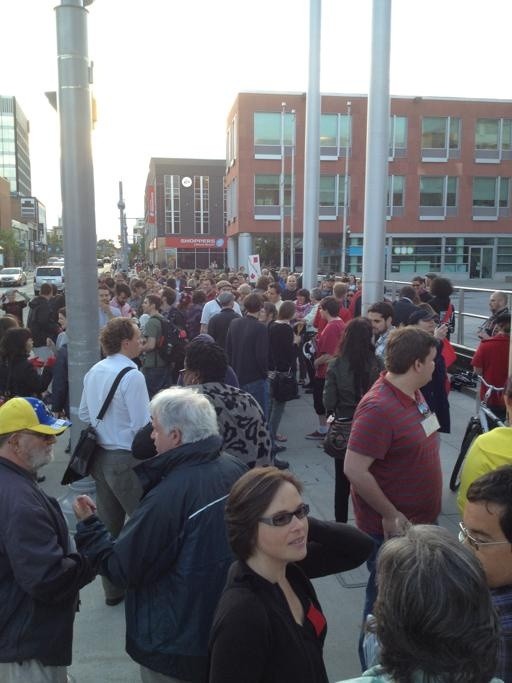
[[61, 367, 138, 485], [270, 346, 300, 402], [323, 361, 384, 458]]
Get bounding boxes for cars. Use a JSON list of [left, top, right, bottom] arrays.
[[0, 267, 27, 286], [103, 257, 110, 263], [48, 257, 59, 265], [34, 266, 65, 296], [97, 259, 104, 268], [53, 258, 64, 266]]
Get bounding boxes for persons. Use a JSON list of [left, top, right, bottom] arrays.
[[1, 395, 108, 678], [0, 279, 69, 414]]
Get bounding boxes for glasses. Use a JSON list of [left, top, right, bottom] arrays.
[[413, 284, 420, 287], [457, 520, 508, 550], [18, 430, 66, 439], [257, 503, 311, 528]]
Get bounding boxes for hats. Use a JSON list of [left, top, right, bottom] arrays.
[[419, 302, 439, 322], [0, 396, 73, 435], [216, 280, 232, 289]]
